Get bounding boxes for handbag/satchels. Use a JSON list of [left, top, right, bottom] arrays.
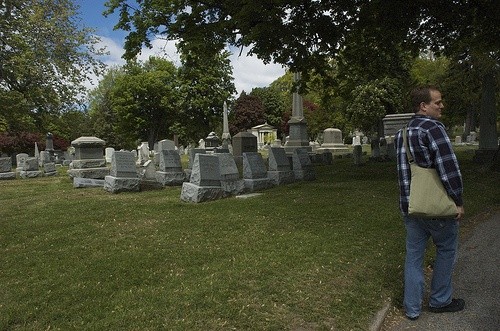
[[408, 162, 458, 218]]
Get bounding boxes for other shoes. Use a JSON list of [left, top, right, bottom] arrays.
[[428, 298, 465, 313]]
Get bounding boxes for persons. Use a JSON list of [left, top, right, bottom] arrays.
[[394, 84, 466, 320]]
